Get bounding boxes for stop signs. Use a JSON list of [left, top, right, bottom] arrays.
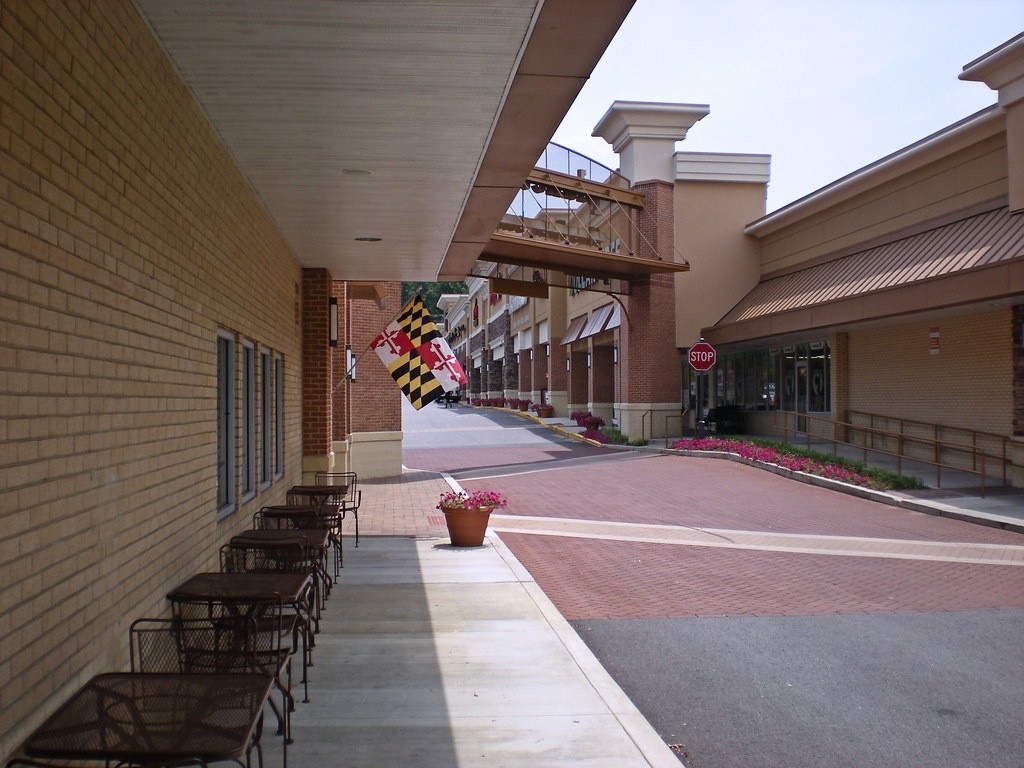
[[688, 342, 717, 371]]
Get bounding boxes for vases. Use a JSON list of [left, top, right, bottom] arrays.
[[587, 424, 598, 432], [444, 508, 490, 547], [474, 402, 552, 417]]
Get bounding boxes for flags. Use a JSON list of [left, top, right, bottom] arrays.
[[371, 294, 468, 411]]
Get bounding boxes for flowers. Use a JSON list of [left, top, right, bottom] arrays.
[[585, 416, 602, 425], [472, 397, 551, 412], [436, 489, 507, 513]]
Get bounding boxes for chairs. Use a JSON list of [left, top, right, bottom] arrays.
[[5, 471, 361, 768]]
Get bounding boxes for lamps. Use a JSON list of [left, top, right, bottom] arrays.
[[345, 344, 352, 379], [351, 352, 356, 381], [329, 296, 339, 347]]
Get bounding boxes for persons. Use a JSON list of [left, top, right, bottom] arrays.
[[445, 392, 452, 408]]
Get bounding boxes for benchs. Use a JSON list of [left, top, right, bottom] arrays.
[[695, 404, 745, 437]]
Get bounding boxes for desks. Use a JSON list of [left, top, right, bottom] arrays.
[[290, 485, 349, 555], [264, 505, 341, 576], [21, 671, 273, 768], [166, 571, 316, 702], [237, 529, 331, 610]]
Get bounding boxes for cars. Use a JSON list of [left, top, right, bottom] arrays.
[[434, 388, 459, 403]]
